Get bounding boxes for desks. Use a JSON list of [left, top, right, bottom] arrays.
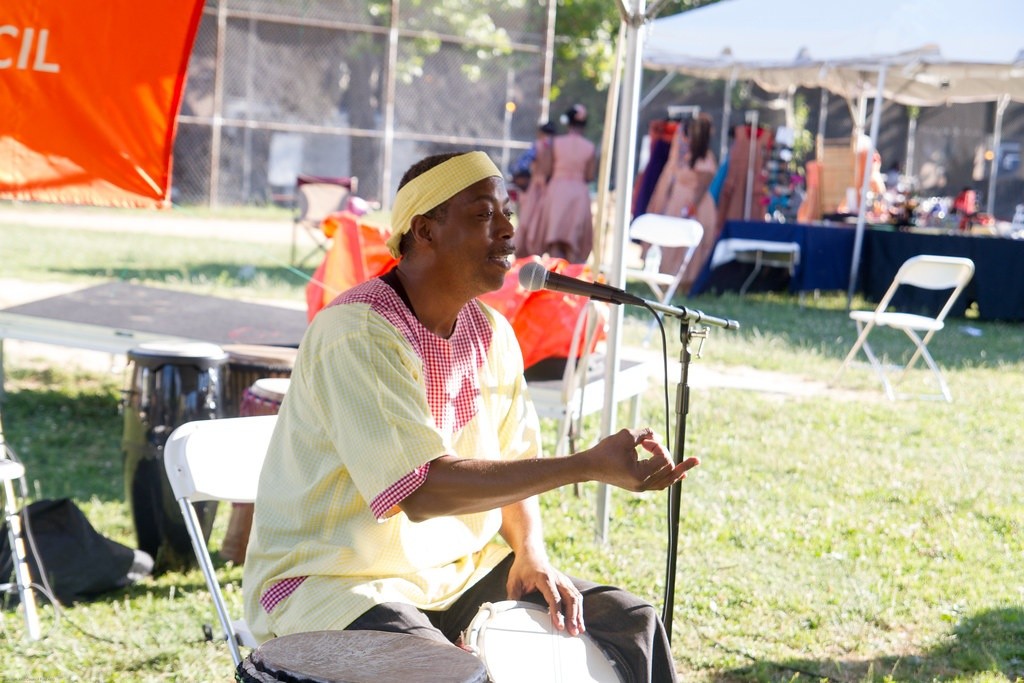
[[0, 279, 648, 570], [694, 219, 1024, 323]]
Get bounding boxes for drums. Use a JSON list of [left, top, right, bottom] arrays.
[[219, 378, 296, 565], [127, 340, 228, 572], [221, 343, 300, 427]]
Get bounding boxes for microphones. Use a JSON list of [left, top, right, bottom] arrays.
[[518, 262, 644, 306]]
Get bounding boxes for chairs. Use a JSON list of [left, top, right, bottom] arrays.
[[291, 175, 360, 268], [823, 253, 976, 402], [164, 411, 277, 666], [630, 212, 704, 331]]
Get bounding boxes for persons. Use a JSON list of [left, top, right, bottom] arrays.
[[508, 123, 556, 258], [241, 143, 699, 683], [506, 168, 532, 206], [527, 103, 597, 263]]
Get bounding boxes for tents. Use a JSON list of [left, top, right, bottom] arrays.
[[595, 0, 1024, 323]]
